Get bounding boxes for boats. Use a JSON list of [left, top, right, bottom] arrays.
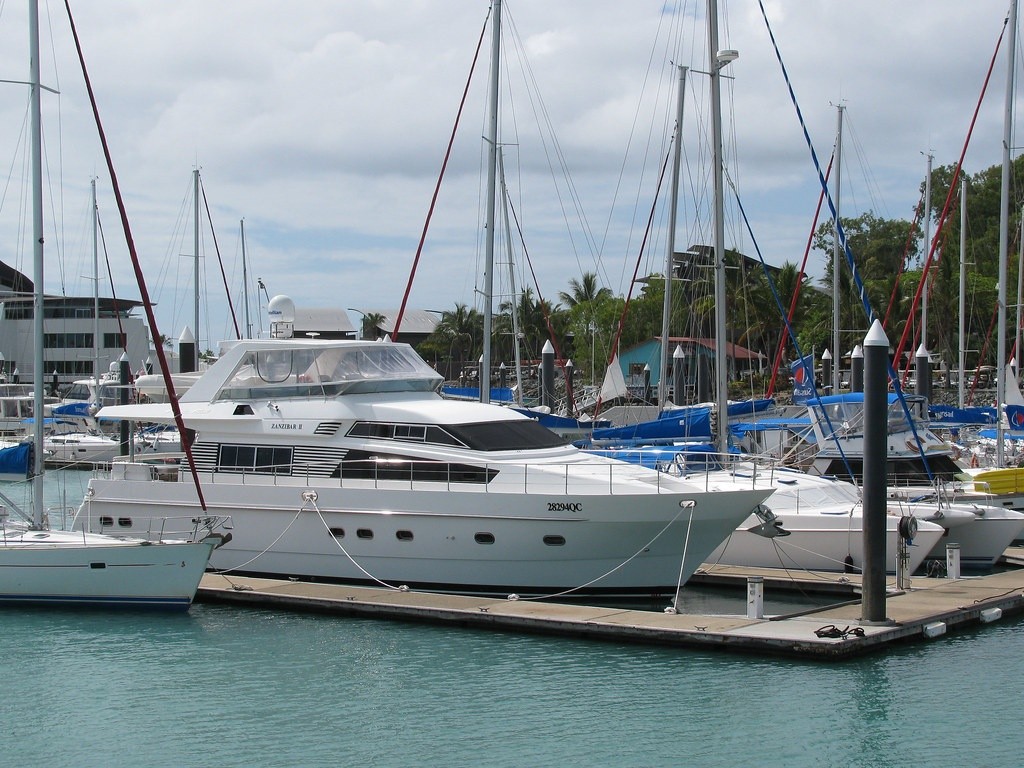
[[67, 293, 779, 614]]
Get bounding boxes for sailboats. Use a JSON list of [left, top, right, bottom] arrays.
[[0, 1, 1024, 583], [0, 1, 231, 616]]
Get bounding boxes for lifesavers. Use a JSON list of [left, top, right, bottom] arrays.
[[947, 445, 961, 461], [904, 434, 927, 453], [970, 453, 979, 468]]
